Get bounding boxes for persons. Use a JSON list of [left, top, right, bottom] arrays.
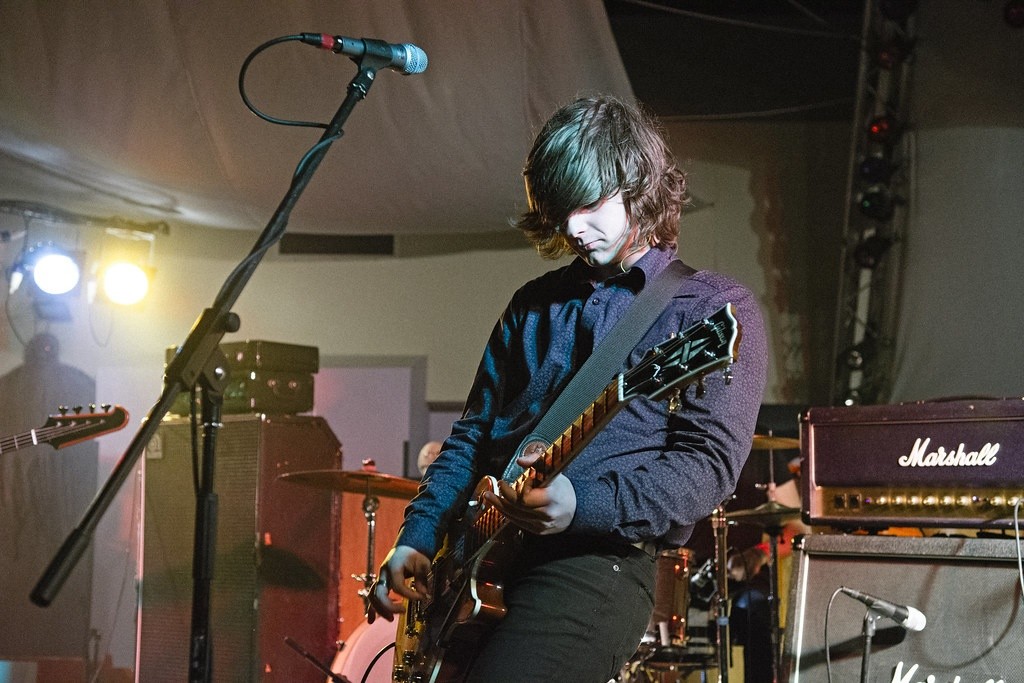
[[367, 96, 768, 683]]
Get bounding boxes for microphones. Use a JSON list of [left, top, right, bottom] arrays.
[[299, 32, 429, 77], [840, 585, 926, 633]]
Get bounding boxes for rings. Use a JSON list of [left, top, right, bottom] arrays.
[[375, 580, 391, 595]]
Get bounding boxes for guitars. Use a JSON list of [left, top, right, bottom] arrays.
[[391, 300, 744, 683], [0, 403, 128, 452]]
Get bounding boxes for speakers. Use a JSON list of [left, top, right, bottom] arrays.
[[127, 415, 344, 683], [779, 532, 1024, 683]]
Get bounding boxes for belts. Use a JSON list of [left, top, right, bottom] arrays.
[[631, 540, 658, 559]]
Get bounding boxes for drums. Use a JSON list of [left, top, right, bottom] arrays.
[[320, 610, 398, 683], [636, 544, 693, 656]]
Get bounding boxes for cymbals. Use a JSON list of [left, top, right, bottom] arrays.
[[274, 465, 420, 499], [751, 434, 800, 449], [723, 498, 803, 525]]
